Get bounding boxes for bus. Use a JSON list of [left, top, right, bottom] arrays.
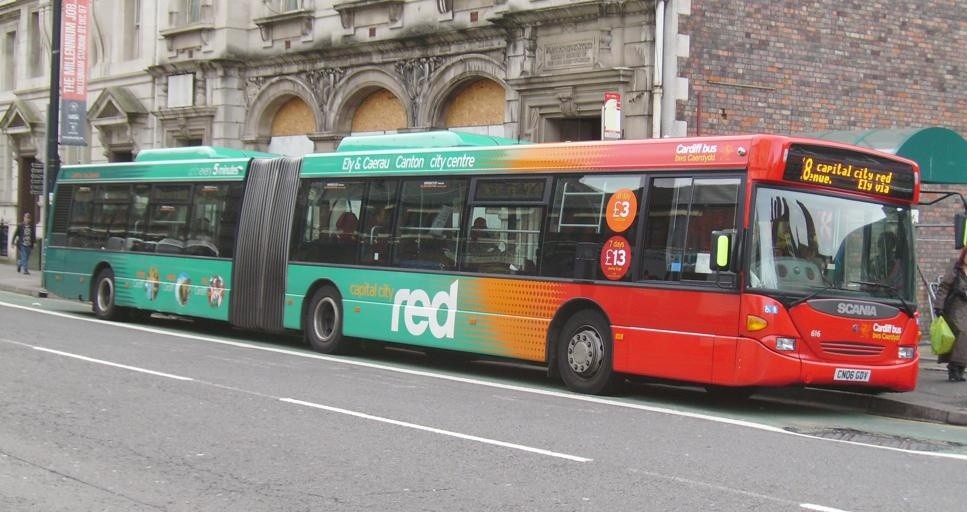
[[40, 131, 966, 405]]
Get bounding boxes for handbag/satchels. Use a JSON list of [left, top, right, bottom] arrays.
[[929, 315, 955, 355]]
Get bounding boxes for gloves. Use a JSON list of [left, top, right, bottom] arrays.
[[935, 310, 943, 317]]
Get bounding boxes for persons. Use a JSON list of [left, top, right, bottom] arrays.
[[931, 245, 966, 382], [862, 232, 898, 285], [358, 207, 387, 260], [10, 211, 36, 277]]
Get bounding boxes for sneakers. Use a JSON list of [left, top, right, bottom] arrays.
[[17, 264, 29, 274]]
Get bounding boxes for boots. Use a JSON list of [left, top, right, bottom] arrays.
[[947, 364, 966, 382]]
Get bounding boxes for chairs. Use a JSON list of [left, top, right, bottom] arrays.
[[335, 213, 359, 239], [470, 218, 502, 257], [107, 236, 219, 257]]
